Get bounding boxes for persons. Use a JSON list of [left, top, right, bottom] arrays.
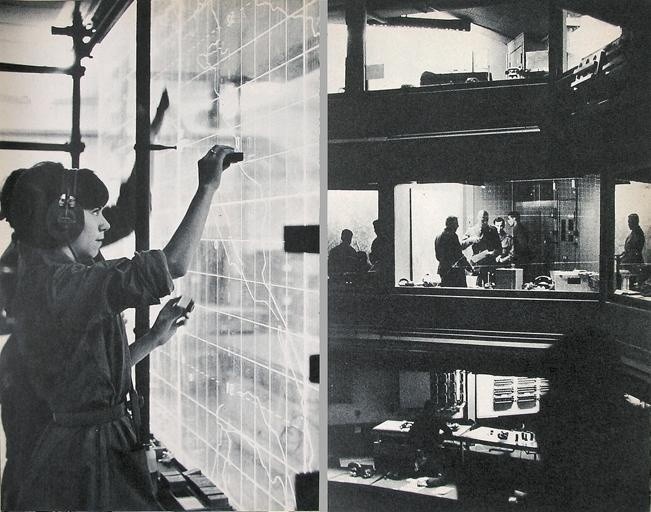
[[622, 214, 645, 262], [1, 91, 167, 336], [410, 401, 452, 453], [368, 220, 379, 264], [435, 208, 526, 286], [330, 229, 356, 274], [1, 145, 243, 512]]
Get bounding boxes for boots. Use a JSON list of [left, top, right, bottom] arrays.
[[427, 444, 460, 487]]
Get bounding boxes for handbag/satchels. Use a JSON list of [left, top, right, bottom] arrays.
[[457, 452, 514, 507], [373, 439, 415, 479], [126, 443, 158, 496]]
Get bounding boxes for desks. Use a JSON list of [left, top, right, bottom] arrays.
[[461, 425, 539, 464], [372, 419, 472, 447]]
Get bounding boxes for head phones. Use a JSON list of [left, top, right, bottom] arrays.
[[47, 168, 85, 246]]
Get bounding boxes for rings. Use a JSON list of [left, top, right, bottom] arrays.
[[210, 149, 216, 154]]
